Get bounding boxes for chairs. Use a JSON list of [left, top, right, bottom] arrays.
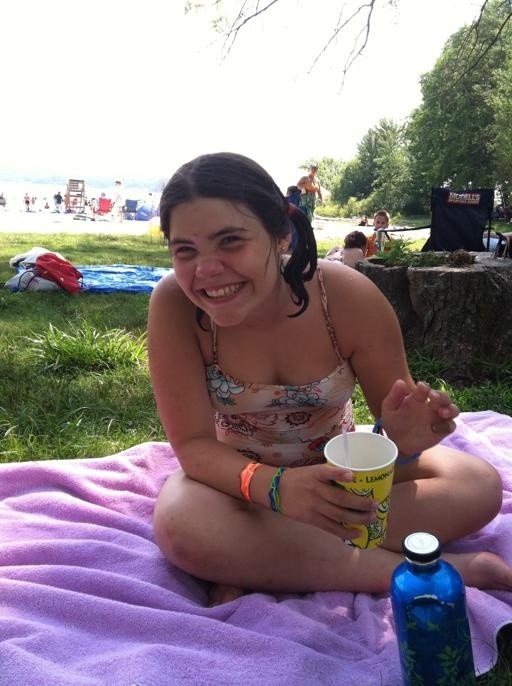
[[379, 187, 507, 256]]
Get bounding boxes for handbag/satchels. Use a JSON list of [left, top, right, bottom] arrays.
[[3, 246, 83, 297]]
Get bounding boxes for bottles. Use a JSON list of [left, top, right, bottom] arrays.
[[388, 530, 477, 684]]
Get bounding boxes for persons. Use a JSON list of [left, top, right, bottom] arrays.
[[358, 216, 369, 226], [284, 186, 311, 254], [296, 163, 325, 231], [0, 181, 126, 223], [145, 153, 512, 595], [366, 210, 398, 256], [324, 231, 370, 272]]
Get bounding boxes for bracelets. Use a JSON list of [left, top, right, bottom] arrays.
[[372, 417, 421, 465], [239, 461, 263, 505], [268, 464, 287, 514]]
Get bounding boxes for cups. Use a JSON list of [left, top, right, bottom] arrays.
[[323, 431, 401, 550]]
